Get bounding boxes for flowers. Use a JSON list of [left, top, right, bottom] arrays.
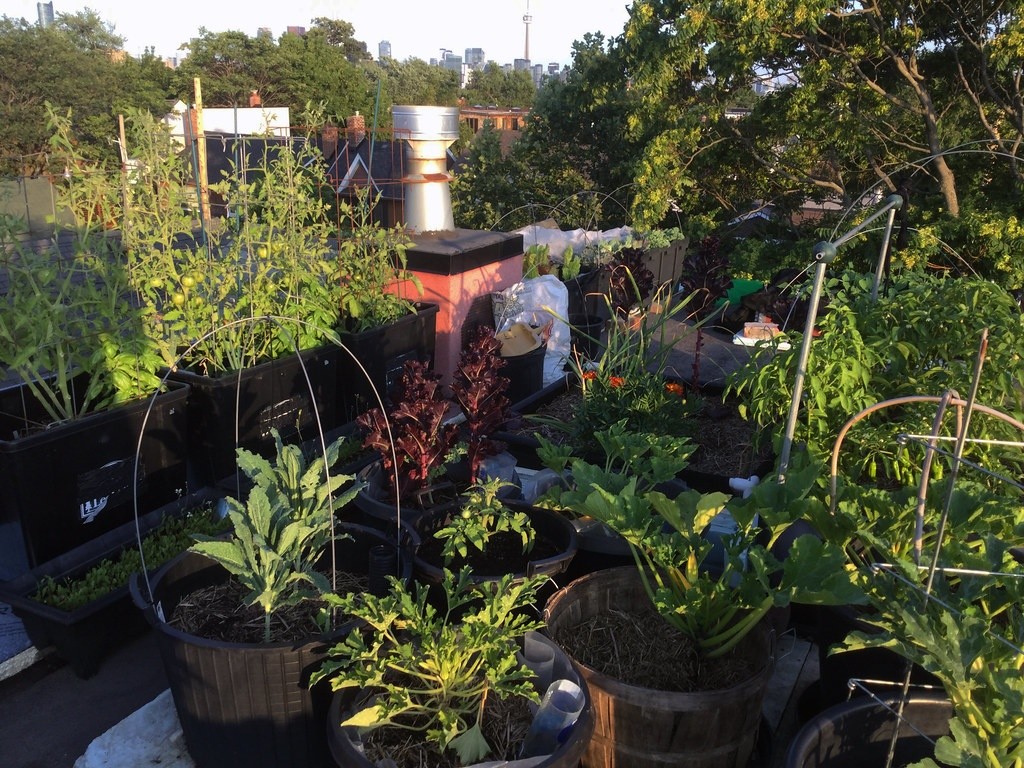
[[539, 265, 730, 431]]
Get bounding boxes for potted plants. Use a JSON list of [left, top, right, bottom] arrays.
[[0, 329, 1024, 768]]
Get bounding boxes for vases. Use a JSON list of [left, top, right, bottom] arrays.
[[501, 362, 776, 497]]
